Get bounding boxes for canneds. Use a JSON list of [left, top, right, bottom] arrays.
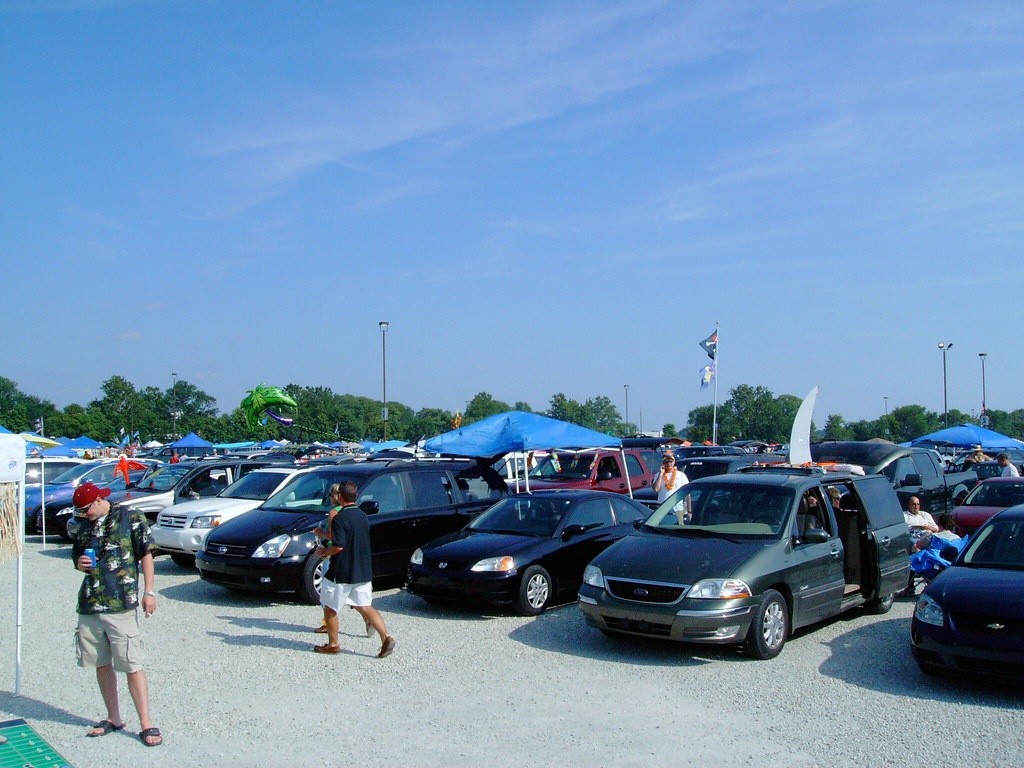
[[84, 548, 97, 569]]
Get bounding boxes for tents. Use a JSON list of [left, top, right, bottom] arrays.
[[416, 409, 633, 499], [312, 440, 408, 455], [19, 432, 291, 459], [681, 440, 713, 446], [911, 422, 1024, 476], [0, 426, 25, 698]]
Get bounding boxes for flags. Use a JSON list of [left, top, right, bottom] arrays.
[[114, 427, 140, 447], [35, 419, 43, 433], [699, 361, 716, 391], [699, 330, 717, 360]]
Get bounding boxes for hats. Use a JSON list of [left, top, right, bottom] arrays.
[[974, 445, 982, 450], [73, 484, 112, 506], [663, 455, 674, 461]]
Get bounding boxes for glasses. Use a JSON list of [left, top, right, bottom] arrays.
[[75, 503, 93, 513]]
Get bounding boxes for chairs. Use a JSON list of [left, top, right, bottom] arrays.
[[775, 493, 808, 539], [101, 470, 112, 482], [707, 488, 756, 526], [451, 479, 469, 503], [211, 475, 227, 494], [373, 477, 400, 513]]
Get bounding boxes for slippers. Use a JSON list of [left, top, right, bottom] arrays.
[[139, 728, 161, 745], [87, 720, 125, 738]]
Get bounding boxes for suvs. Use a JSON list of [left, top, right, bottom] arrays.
[[576, 462, 911, 661], [150, 456, 402, 573], [194, 449, 521, 606]]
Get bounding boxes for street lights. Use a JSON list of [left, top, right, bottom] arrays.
[[623, 384, 630, 426], [172, 372, 178, 441], [979, 353, 987, 427], [378, 321, 391, 442], [883, 397, 888, 418], [938, 341, 954, 456]]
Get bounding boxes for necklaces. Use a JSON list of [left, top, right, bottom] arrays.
[[84, 499, 113, 561], [663, 467, 676, 490]]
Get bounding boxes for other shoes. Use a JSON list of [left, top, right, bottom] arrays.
[[365, 616, 376, 636], [378, 637, 395, 657], [314, 644, 340, 653], [314, 626, 328, 632], [899, 588, 915, 596]]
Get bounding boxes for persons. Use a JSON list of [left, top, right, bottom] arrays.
[[170, 452, 189, 463], [652, 449, 692, 525], [807, 486, 841, 508], [313, 479, 395, 657], [997, 453, 1020, 477], [71, 483, 162, 746], [966, 445, 990, 462], [897, 496, 961, 596], [83, 451, 91, 459]]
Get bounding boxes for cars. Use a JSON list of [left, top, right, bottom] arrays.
[[442, 435, 1023, 515], [909, 505, 1024, 679], [15, 445, 371, 560], [943, 476, 1024, 538], [405, 488, 680, 617]]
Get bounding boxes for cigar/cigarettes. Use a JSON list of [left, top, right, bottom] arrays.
[[148, 613, 152, 616]]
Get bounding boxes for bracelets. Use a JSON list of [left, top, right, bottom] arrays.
[[688, 511, 692, 513], [145, 591, 156, 597]]
